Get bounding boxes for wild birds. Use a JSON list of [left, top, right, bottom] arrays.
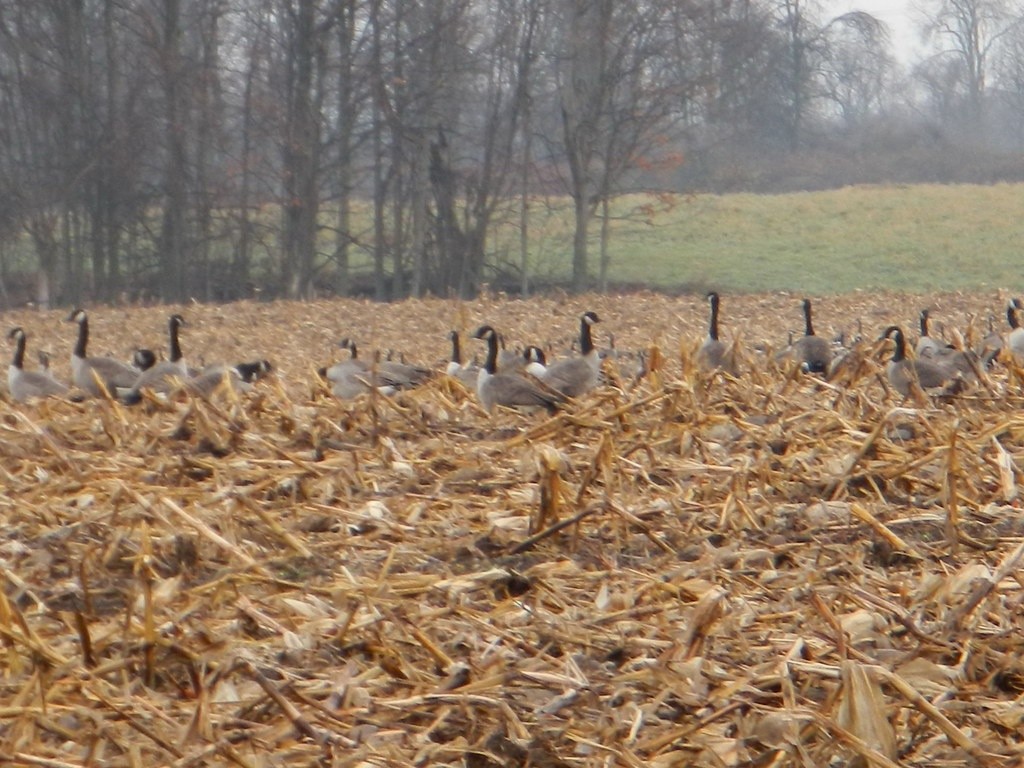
[[695, 292, 740, 378], [318, 312, 606, 423], [63, 309, 271, 413], [791, 297, 1024, 399], [6, 328, 71, 403]]
